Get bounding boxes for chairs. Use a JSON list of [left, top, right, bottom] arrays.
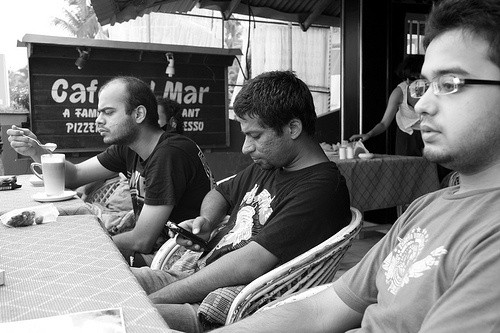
[[151, 205, 362, 326], [71, 176, 142, 206]]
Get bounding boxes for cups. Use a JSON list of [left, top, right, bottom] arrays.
[[346, 147, 354, 160], [339, 147, 345, 160], [30, 154, 65, 197]]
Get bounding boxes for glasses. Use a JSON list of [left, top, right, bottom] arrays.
[[409, 75, 500, 98]]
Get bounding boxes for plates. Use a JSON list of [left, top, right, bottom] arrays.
[[32, 191, 77, 201], [358, 153, 374, 159]]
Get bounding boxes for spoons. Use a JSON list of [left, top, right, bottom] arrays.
[[23, 135, 57, 149]]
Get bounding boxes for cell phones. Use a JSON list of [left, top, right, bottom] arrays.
[[165, 221, 212, 252]]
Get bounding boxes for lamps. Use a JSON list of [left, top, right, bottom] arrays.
[[166, 50, 174, 77], [74, 47, 95, 70]]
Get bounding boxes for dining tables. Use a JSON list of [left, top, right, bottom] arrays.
[[320, 154, 443, 219], [0, 174, 173, 333]]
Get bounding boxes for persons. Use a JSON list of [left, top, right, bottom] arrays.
[[6, 76, 217, 267], [349, 54, 425, 156], [129, 70, 352, 332], [74, 98, 185, 234], [205, 0, 500, 333]]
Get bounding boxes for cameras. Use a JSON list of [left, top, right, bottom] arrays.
[[0, 176, 17, 191]]
